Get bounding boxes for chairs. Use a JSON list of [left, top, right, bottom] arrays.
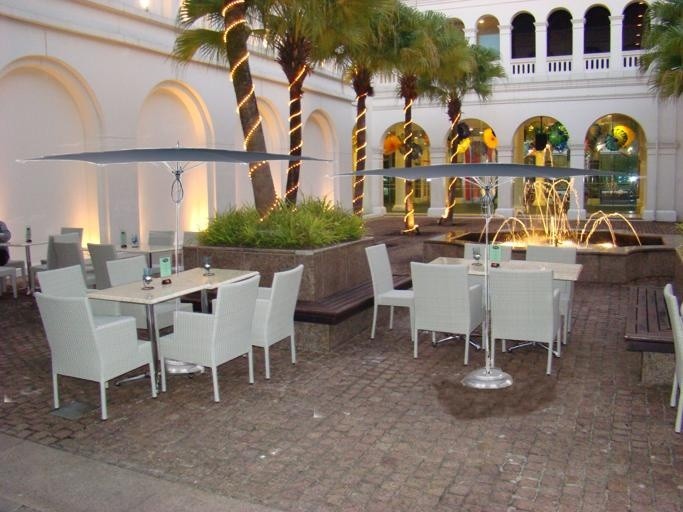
[[33, 295, 157, 422], [1, 266, 19, 298], [364, 241, 583, 382], [158, 278, 258, 404], [52, 241, 87, 263], [150, 230, 175, 240], [86, 242, 116, 257], [663, 283, 683, 434], [258, 265, 305, 386], [101, 258, 153, 283], [35, 265, 94, 296]]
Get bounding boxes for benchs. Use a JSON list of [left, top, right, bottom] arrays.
[[624, 284, 682, 387], [182, 272, 412, 325]]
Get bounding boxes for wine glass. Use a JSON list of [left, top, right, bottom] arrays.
[[143, 267, 154, 290], [203, 256, 214, 276], [472, 247, 481, 267]]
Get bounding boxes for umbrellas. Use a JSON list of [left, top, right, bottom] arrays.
[[14, 139, 335, 335], [328, 148, 643, 373]]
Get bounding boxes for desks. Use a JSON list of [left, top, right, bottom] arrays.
[[118, 239, 183, 260], [6, 240, 42, 290], [94, 269, 257, 322]]
